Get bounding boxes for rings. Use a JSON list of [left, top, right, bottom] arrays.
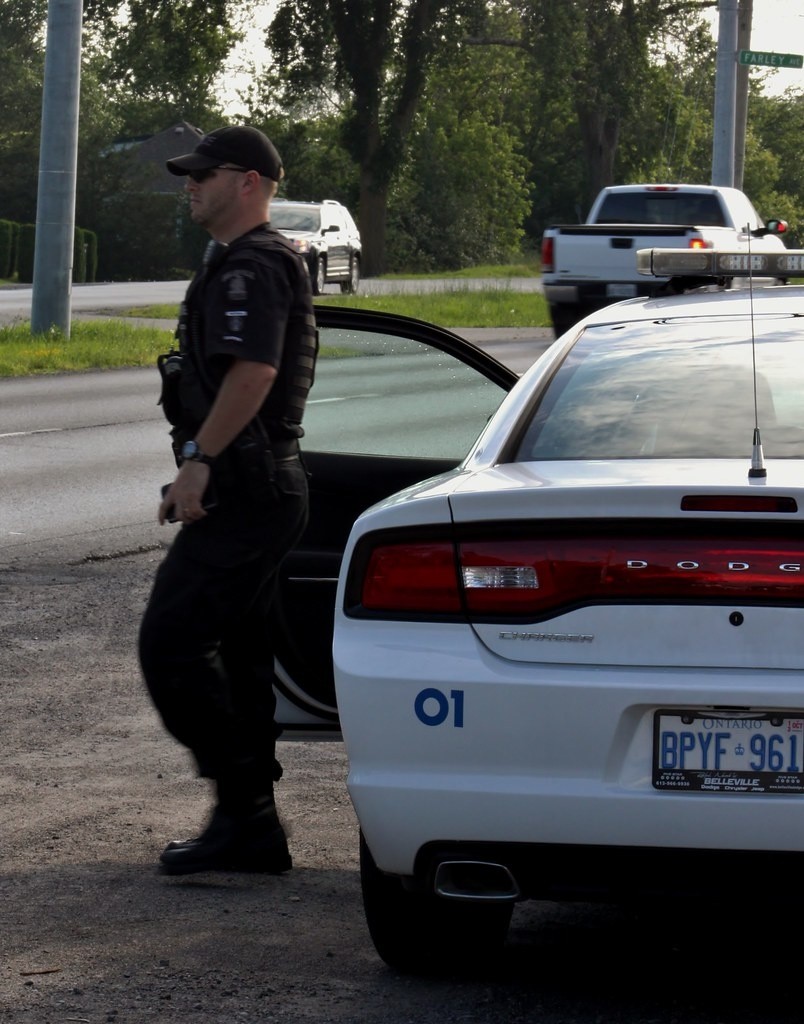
[[182, 508, 190, 512]]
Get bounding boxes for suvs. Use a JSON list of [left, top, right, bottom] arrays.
[[203, 200, 365, 297]]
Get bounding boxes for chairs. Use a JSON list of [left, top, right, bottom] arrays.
[[610, 364, 775, 456]]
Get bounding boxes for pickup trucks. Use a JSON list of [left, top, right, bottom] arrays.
[[537, 183, 792, 339]]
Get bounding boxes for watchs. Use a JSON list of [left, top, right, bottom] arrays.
[[138, 129, 318, 875], [182, 441, 215, 467]]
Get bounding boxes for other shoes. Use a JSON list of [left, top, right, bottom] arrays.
[[161, 799, 291, 874]]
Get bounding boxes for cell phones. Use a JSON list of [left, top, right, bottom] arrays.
[[162, 478, 220, 523]]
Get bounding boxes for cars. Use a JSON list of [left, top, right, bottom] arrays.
[[260, 243, 799, 976]]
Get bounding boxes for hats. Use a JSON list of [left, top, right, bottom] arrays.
[[164, 125, 283, 182]]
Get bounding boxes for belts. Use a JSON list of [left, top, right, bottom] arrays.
[[216, 439, 301, 473]]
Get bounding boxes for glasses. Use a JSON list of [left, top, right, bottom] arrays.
[[188, 167, 265, 184]]
